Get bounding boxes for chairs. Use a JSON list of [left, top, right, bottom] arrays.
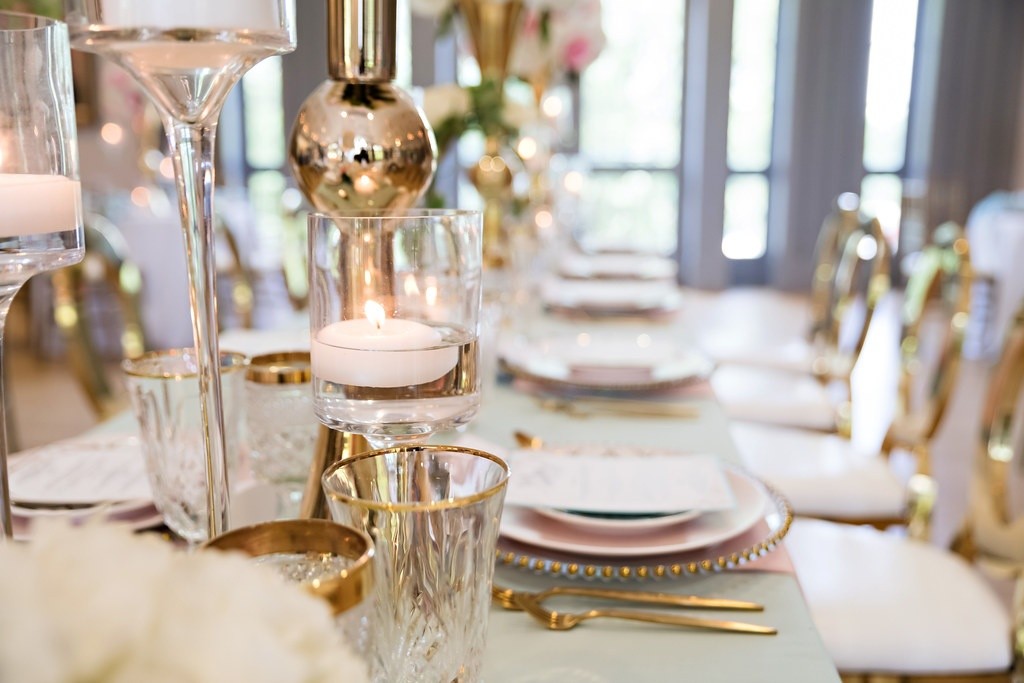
[[52, 216, 151, 420], [701, 194, 868, 373], [710, 217, 889, 439], [765, 307, 1024, 683], [732, 238, 971, 539]]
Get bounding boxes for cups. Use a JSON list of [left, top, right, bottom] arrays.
[[1, 348, 507, 683]]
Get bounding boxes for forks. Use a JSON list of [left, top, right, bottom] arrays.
[[492, 584, 765, 611], [513, 595, 778, 635]]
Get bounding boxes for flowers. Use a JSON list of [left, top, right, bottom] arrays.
[[397, 0, 607, 203]]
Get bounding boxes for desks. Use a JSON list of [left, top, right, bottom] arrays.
[[0, 239, 847, 683]]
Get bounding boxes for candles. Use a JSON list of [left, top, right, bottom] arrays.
[[0, 7, 86, 539], [65, 0, 296, 541], [306, 207, 485, 514]]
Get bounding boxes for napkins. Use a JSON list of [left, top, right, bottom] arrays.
[[500, 445, 738, 514]]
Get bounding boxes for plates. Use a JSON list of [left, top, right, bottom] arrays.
[[531, 505, 701, 536], [497, 470, 792, 580], [499, 356, 710, 392]]
